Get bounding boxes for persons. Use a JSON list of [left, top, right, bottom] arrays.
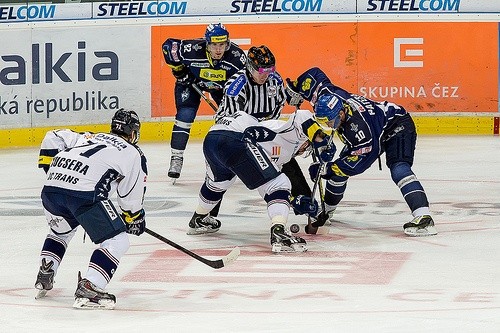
[[162, 23, 247, 185], [35, 108, 148, 310], [209, 45, 318, 210], [187, 110, 337, 255], [285, 67, 438, 237]]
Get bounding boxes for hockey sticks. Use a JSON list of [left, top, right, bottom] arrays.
[[285, 77, 326, 233], [305, 129, 336, 235], [191, 82, 219, 112], [142, 227, 241, 270]]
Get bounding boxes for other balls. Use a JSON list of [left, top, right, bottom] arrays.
[[290, 223, 300, 234]]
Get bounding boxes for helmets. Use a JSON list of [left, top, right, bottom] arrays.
[[295, 140, 313, 159], [245, 44, 275, 74], [204, 23, 230, 46], [111, 108, 141, 144], [313, 93, 344, 123]]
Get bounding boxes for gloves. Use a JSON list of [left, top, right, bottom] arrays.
[[121, 209, 146, 236], [291, 194, 319, 217], [171, 67, 196, 85], [313, 136, 336, 163], [308, 161, 335, 183]]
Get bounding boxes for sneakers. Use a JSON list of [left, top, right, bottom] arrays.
[[316, 204, 337, 226], [73, 271, 117, 310], [34, 258, 55, 299], [403, 215, 437, 237], [186, 211, 221, 235], [168, 155, 183, 185], [270, 224, 308, 254]]
